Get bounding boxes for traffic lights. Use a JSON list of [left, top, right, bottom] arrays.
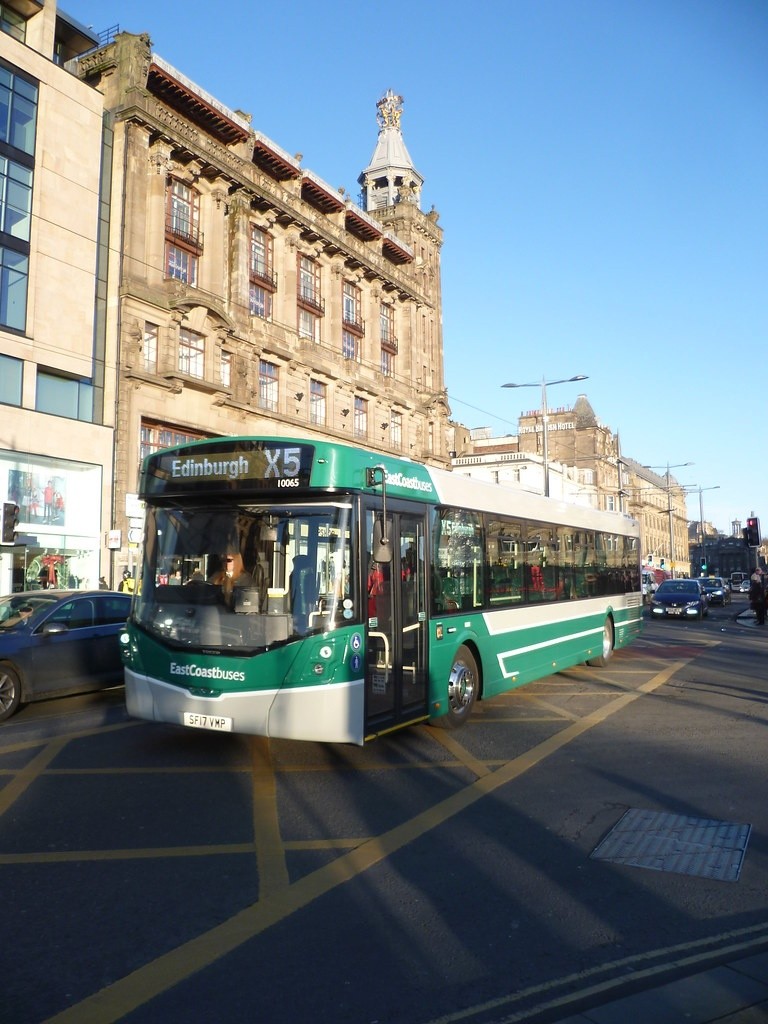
[[739, 527, 750, 548], [659, 558, 665, 569], [0, 501, 20, 546], [647, 556, 652, 566], [746, 517, 762, 547], [700, 556, 707, 571]]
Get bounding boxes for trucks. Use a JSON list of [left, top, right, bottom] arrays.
[[641, 570, 659, 605]]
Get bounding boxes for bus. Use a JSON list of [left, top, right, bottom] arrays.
[[118, 434, 644, 748], [730, 572, 749, 591]]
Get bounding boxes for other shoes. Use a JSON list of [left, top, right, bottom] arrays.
[[756, 622, 764, 626], [754, 620, 760, 623]]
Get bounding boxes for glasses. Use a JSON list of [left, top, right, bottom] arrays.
[[222, 559, 234, 563]]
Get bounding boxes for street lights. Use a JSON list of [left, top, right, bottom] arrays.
[[683, 485, 720, 577], [640, 460, 695, 579], [499, 374, 589, 497]]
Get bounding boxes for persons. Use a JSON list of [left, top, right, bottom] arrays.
[[192, 568, 204, 581], [28, 479, 63, 523], [99, 577, 109, 590], [208, 549, 254, 605], [11, 605, 33, 629], [157, 569, 181, 585], [748, 567, 765, 625], [118, 570, 135, 595]]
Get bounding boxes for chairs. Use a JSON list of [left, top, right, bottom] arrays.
[[69, 602, 92, 628], [433, 562, 596, 612]]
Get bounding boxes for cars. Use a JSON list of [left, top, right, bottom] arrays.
[[694, 575, 732, 608], [739, 580, 750, 593], [647, 578, 709, 620], [0, 588, 142, 722]]
